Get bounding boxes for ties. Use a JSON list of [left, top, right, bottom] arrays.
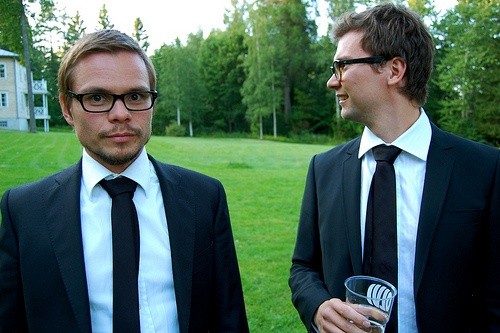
[[99, 175, 141, 333], [364, 142, 402, 333]]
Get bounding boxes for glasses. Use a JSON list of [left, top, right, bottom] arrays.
[[330, 56, 392, 81], [65, 89, 158, 113]]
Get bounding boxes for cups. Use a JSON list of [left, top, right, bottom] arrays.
[[344, 276, 397, 333]]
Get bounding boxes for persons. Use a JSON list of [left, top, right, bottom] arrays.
[[289, 2, 500, 333], [1, 29, 254, 332]]
